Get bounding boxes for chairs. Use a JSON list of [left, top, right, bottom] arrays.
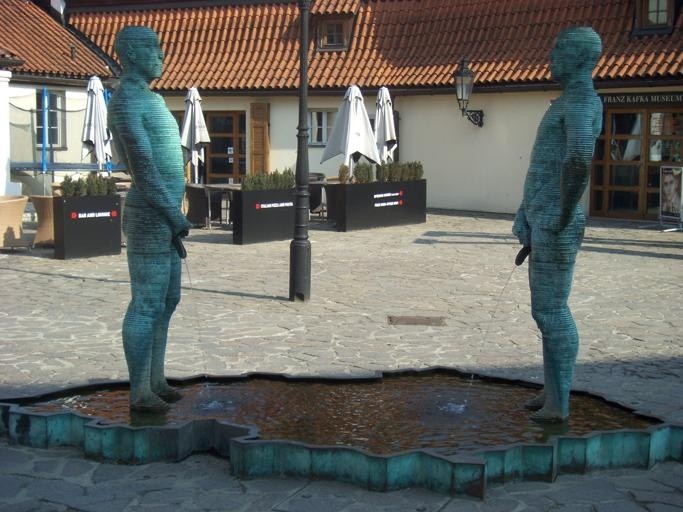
[[0, 195, 29, 250], [307, 173, 325, 212], [183, 181, 224, 225], [30, 194, 58, 248]]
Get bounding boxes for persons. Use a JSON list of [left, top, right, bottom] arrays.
[[662, 172, 681, 215], [508, 24, 605, 425], [102, 25, 188, 414]]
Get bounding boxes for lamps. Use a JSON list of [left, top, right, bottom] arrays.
[[451, 54, 484, 129]]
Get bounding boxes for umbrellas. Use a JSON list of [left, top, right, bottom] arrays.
[[372, 82, 401, 168], [318, 85, 383, 181], [178, 86, 211, 183], [80, 74, 112, 166]]
